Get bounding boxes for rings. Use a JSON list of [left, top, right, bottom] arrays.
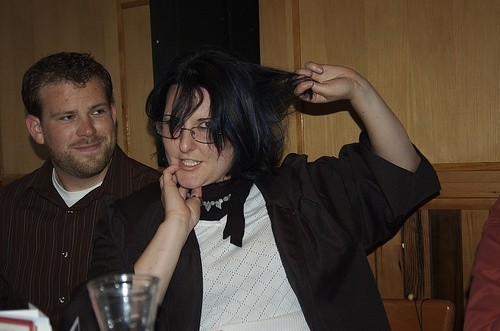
[[186, 193, 203, 201]]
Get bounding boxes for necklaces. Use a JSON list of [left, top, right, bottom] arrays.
[[201, 194, 232, 212]]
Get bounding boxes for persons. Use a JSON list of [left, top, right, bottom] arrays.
[[463, 195, 500, 331], [0, 52, 163, 331], [60, 43, 441, 331]]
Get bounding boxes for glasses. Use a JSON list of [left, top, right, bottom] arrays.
[[156, 115, 223, 144]]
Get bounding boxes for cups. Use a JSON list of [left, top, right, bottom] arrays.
[[87, 274, 160, 331]]
